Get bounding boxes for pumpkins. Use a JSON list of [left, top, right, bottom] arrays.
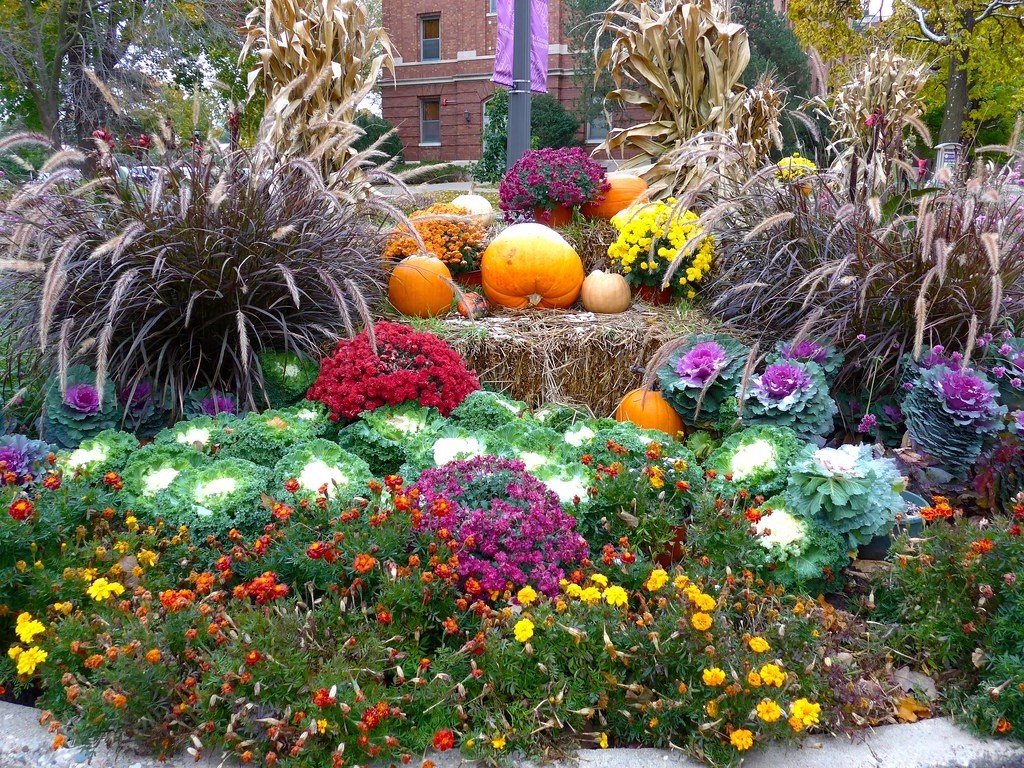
[[458, 292, 491, 317], [451, 181, 492, 229], [582, 268, 630, 314], [387, 249, 454, 318], [580, 174, 651, 219], [482, 223, 585, 309], [615, 381, 686, 443]]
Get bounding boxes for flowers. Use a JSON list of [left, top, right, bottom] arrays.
[[497, 146, 611, 223], [774, 153, 819, 186], [378, 195, 490, 275], [604, 196, 715, 299]]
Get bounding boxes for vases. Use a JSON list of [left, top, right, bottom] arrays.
[[533, 202, 573, 227], [451, 269, 483, 291], [633, 283, 673, 306]]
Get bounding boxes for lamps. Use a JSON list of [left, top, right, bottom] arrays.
[[464, 108, 471, 122]]
